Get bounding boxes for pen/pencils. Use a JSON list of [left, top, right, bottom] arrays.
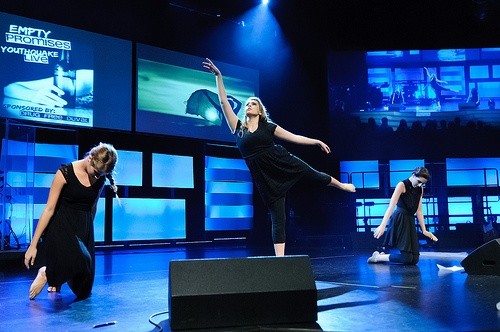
[[92, 321, 115, 328]]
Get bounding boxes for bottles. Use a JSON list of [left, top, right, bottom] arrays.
[[54, 36, 76, 109]]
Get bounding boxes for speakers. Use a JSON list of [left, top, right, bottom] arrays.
[[0, 249, 28, 269], [459, 101, 476, 110], [389, 104, 406, 112], [168, 255, 317, 332], [461, 238, 500, 275]]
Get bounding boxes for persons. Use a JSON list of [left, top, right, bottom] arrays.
[[392, 90, 404, 105], [466, 88, 481, 109], [202, 58, 356, 256], [24, 144, 121, 300], [3, 69, 93, 108], [424, 66, 459, 109], [199, 91, 242, 134], [367, 167, 438, 266]]
